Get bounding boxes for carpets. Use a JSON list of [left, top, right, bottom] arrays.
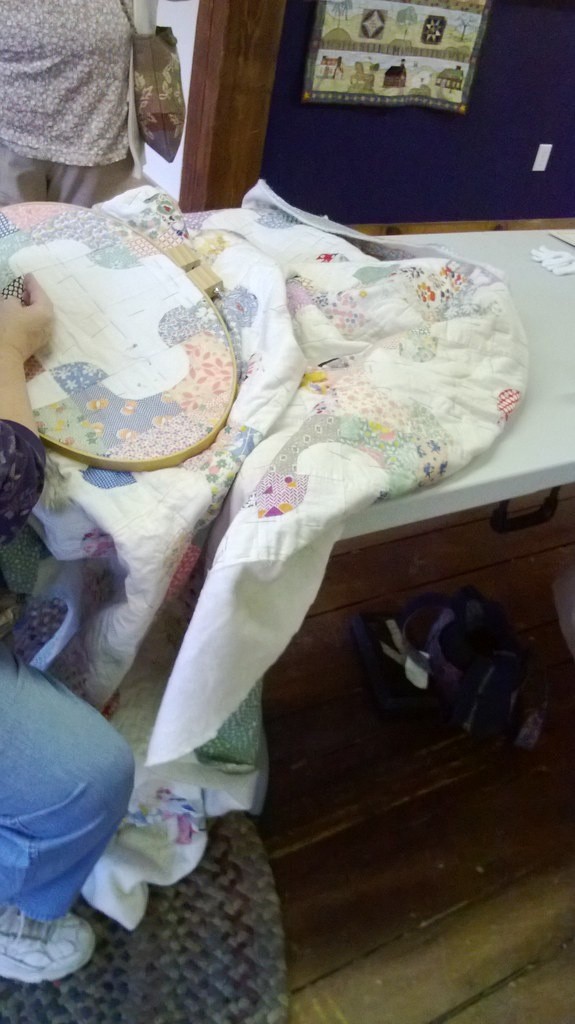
[[0, 802, 290, 1023]]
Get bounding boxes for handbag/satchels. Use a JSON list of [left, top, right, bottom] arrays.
[[131, 26, 185, 164]]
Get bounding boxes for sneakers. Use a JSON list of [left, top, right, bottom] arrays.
[[0, 906, 96, 985]]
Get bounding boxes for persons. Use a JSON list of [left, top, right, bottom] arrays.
[[0, 0, 159, 209], [0, 273, 135, 984]]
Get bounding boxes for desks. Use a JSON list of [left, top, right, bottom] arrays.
[[211, 228, 575, 582]]
